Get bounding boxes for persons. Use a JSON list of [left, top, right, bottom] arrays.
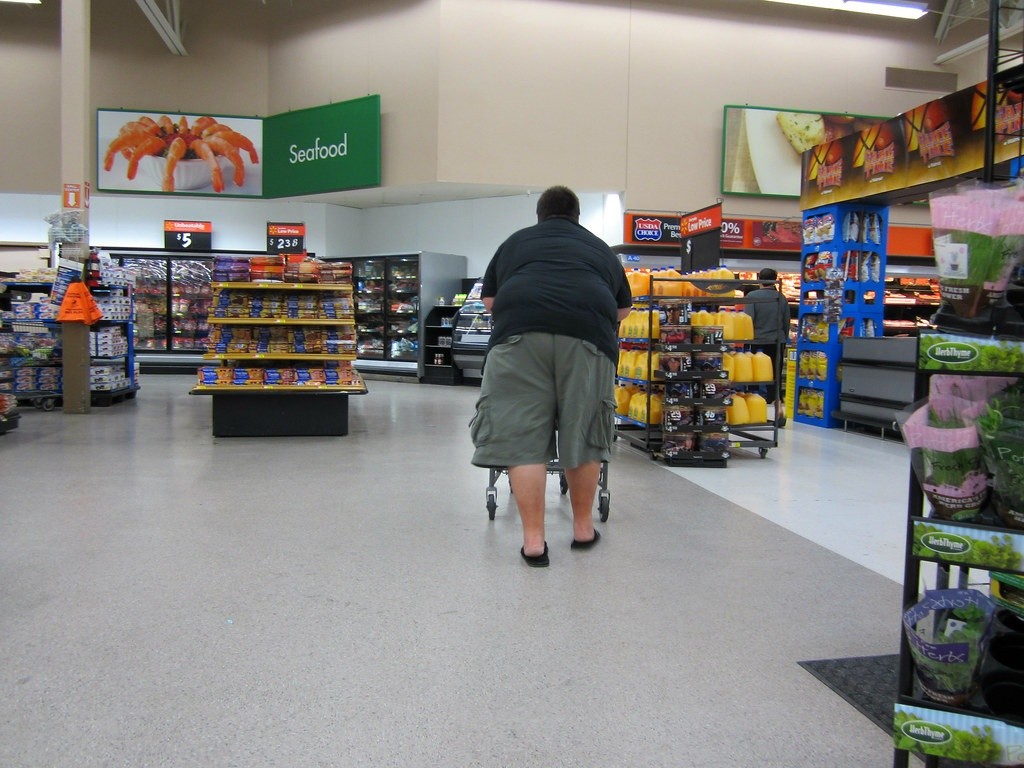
[[742, 268, 791, 428], [467, 185, 635, 569]]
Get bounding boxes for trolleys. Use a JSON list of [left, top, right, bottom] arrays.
[[481, 311, 621, 523]]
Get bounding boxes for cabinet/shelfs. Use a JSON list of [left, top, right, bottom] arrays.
[[0, 279, 782, 460]]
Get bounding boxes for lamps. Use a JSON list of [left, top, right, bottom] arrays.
[[764, 0, 929, 20]]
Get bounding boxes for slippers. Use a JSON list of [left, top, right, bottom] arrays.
[[520, 541, 549, 567], [571, 529, 600, 549], [773, 417, 786, 427]]
